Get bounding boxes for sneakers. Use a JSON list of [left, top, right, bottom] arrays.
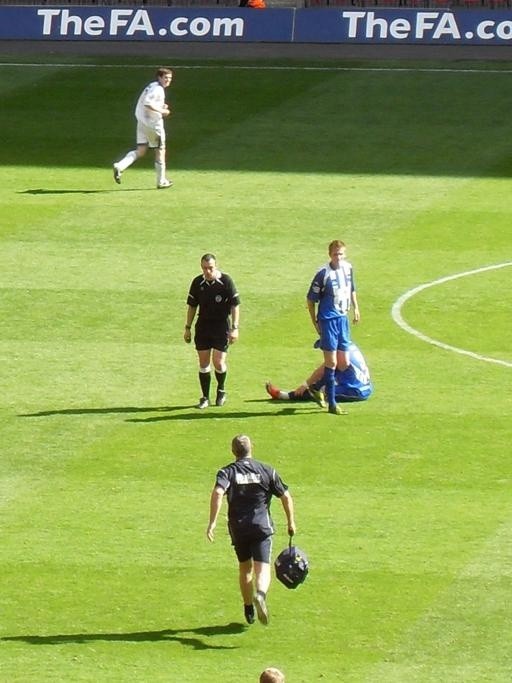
[[113, 163, 123, 184], [266, 382, 281, 400], [157, 180, 173, 189], [216, 397, 226, 406], [307, 383, 327, 408], [196, 398, 209, 408], [254, 595, 269, 625], [244, 610, 255, 624], [328, 405, 347, 415]]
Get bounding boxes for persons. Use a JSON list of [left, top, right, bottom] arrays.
[[307, 240, 360, 415], [206, 435, 296, 625], [114, 69, 174, 188], [184, 253, 241, 409], [266, 339, 372, 401]]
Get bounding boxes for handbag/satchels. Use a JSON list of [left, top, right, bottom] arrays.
[[274, 534, 309, 590]]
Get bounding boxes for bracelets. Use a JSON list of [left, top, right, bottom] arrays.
[[232, 324, 239, 329], [185, 325, 191, 329]]
